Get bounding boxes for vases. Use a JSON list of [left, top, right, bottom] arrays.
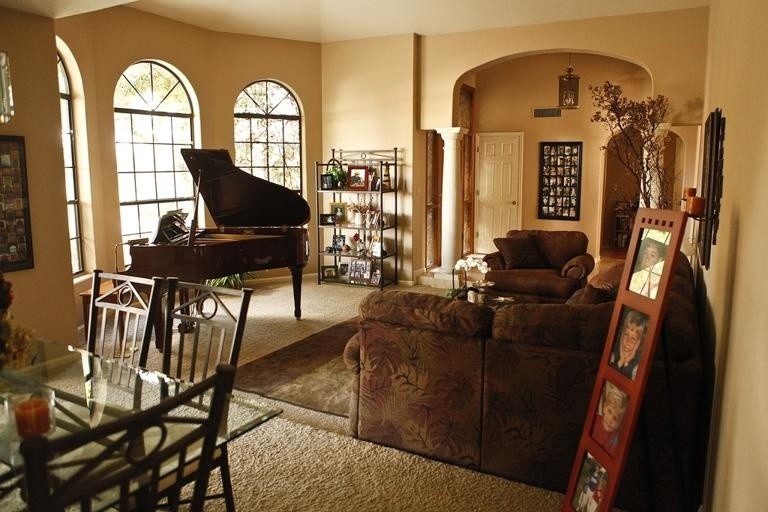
[[354, 213, 367, 225], [357, 244, 363, 252]]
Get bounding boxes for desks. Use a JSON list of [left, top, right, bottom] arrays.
[[0, 329, 284, 512]]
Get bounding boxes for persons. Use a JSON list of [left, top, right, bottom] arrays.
[[541, 145, 579, 217], [609, 311, 647, 381], [575, 465, 601, 512], [350, 172, 365, 187], [590, 390, 631, 456], [638, 239, 661, 269], [336, 207, 342, 216]]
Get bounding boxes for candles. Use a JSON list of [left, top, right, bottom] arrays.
[[684, 188, 697, 201], [687, 195, 706, 217]]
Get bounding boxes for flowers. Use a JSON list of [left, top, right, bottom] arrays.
[[0, 267, 17, 317], [348, 196, 370, 213], [353, 234, 364, 242]]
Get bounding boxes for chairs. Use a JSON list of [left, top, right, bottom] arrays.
[[76, 269, 163, 509], [77, 238, 149, 351], [161, 276, 255, 512]]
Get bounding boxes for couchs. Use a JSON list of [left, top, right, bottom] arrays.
[[343, 248, 716, 512], [483, 229, 596, 304]]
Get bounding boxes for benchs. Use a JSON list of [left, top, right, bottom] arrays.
[[79, 271, 164, 444]]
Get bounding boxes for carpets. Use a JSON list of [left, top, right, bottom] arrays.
[[232, 314, 365, 420]]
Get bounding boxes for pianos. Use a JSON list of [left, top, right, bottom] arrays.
[[111, 149, 311, 354]]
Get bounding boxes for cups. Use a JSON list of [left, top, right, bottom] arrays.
[[5, 386, 56, 441]]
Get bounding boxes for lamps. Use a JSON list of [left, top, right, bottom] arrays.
[[560, 53, 580, 108]]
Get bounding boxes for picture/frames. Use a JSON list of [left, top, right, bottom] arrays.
[[537, 141, 582, 223], [0, 133, 36, 276], [340, 263, 348, 275], [321, 265, 338, 279], [369, 237, 379, 252], [371, 272, 382, 286], [329, 202, 347, 223], [346, 165, 368, 191], [333, 234, 346, 252], [322, 173, 333, 190], [320, 213, 336, 226]]
